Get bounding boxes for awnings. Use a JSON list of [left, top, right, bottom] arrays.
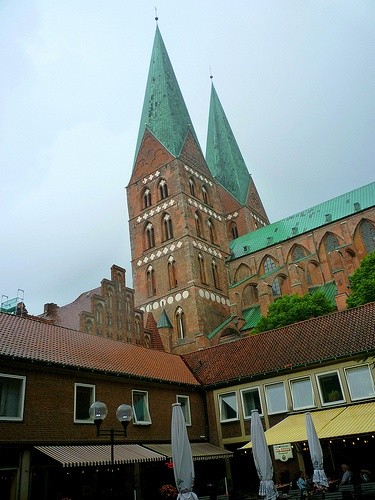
[[33, 442, 168, 466], [141, 440, 235, 461], [236, 406, 347, 449], [316, 401, 375, 440]]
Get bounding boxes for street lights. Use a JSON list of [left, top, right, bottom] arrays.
[[89, 402, 134, 500]]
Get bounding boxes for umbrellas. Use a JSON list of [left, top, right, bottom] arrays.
[[251, 408, 279, 500], [304, 411, 330, 500], [170, 402, 200, 499]]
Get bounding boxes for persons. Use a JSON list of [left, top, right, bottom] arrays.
[[297, 462, 370, 491]]
